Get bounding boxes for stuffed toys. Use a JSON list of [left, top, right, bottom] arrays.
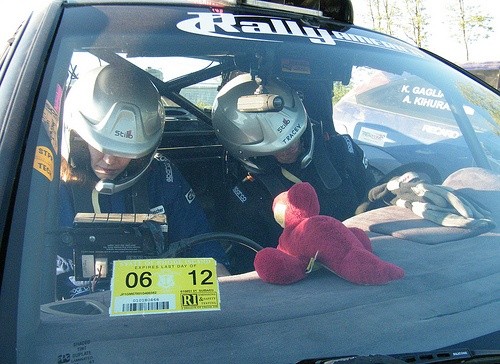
[[254, 182, 406, 286]]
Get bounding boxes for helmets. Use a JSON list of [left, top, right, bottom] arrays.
[[61, 65, 166, 195], [212, 72, 315, 173]]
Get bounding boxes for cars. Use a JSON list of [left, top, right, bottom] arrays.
[[0, 0, 500, 364], [333, 59, 500, 224]]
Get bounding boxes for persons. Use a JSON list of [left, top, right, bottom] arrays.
[[211, 71, 383, 274], [57, 64, 231, 301]]
